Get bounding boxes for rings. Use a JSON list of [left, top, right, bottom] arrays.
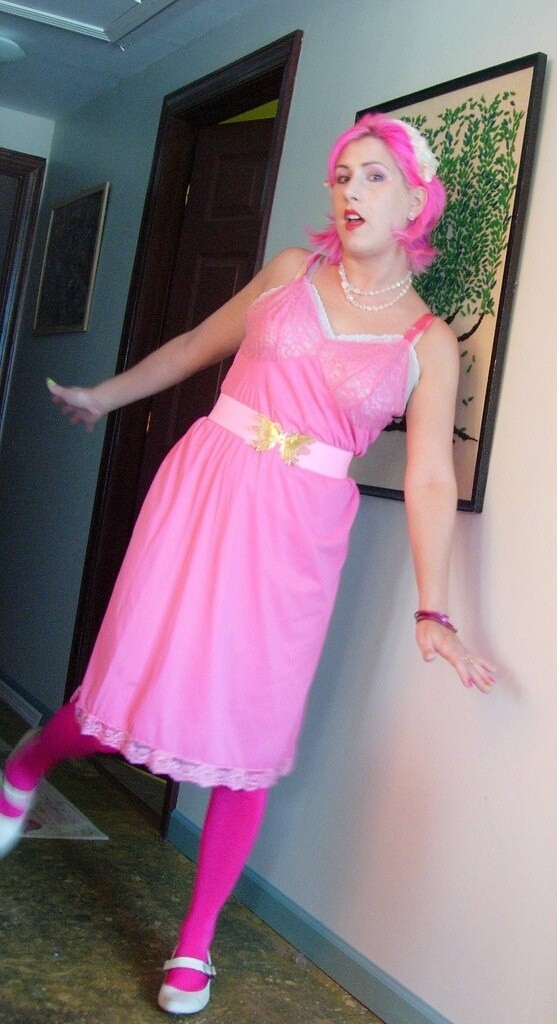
[[471, 660, 473, 662]]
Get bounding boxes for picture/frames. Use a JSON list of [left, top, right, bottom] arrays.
[[349, 51, 548, 513], [31, 180, 110, 337]]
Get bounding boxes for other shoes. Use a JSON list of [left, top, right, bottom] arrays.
[[157, 940, 214, 1013], [0, 726, 45, 859]]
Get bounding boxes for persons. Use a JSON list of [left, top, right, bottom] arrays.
[[0, 113, 502, 1014]]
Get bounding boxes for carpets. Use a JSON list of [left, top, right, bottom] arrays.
[[0, 736, 109, 840]]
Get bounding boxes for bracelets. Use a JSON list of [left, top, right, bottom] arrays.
[[414, 610, 457, 632]]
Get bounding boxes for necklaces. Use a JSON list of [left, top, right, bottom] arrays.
[[339, 262, 413, 310]]
